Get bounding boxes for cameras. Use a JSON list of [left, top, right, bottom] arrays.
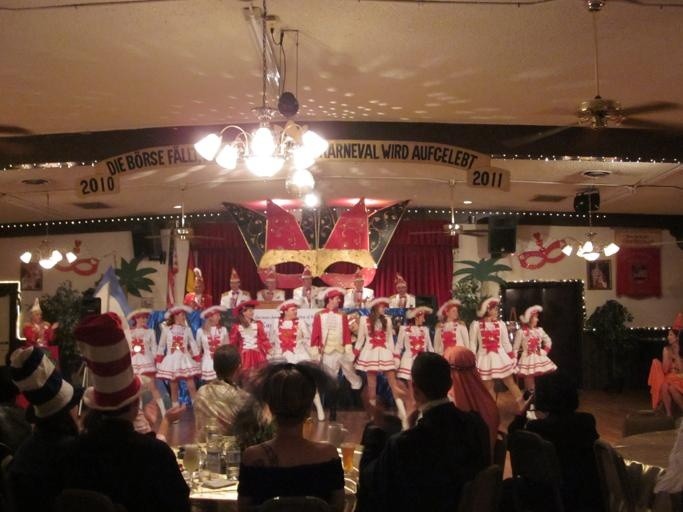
[[524, 391, 531, 400]]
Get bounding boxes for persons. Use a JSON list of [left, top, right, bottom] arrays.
[[0, 362, 32, 453], [44, 396, 193, 511], [503, 371, 599, 510], [358, 350, 491, 510], [436, 345, 503, 464], [658, 324, 682, 422], [0, 400, 86, 511], [116, 264, 558, 432], [20, 306, 61, 371], [227, 358, 347, 511]]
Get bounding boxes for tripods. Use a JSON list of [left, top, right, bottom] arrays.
[[78, 362, 90, 417]]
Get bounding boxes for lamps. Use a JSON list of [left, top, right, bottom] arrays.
[[19, 193, 77, 271], [191, 0, 332, 211], [559, 186, 621, 262]]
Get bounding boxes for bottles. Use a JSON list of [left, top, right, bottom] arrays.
[[202, 423, 241, 481]]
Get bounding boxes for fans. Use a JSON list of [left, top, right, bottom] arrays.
[[143, 182, 227, 242], [500, 0, 682, 152], [408, 178, 490, 238]]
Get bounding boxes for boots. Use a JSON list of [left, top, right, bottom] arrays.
[[328, 392, 337, 421], [313, 394, 326, 421], [395, 397, 410, 431], [171, 402, 181, 423]]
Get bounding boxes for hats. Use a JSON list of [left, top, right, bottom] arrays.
[[126, 304, 227, 319], [70, 312, 143, 410], [353, 268, 364, 282], [302, 267, 312, 279], [6, 345, 86, 424], [230, 268, 240, 284], [232, 298, 261, 316], [265, 269, 277, 283], [436, 298, 543, 323], [194, 268, 203, 284], [672, 314, 683, 331], [395, 274, 407, 288], [277, 299, 302, 313], [30, 297, 41, 313], [318, 288, 433, 319]]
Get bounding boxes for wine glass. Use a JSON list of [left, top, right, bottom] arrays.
[[183, 443, 201, 489]]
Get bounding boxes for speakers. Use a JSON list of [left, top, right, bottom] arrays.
[[574, 193, 599, 214], [133, 222, 162, 260], [81, 297, 101, 320], [489, 220, 516, 254]]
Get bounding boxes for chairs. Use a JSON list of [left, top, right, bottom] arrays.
[[49, 486, 113, 511], [256, 494, 339, 512], [456, 461, 504, 511], [593, 437, 636, 510], [505, 430, 565, 512]]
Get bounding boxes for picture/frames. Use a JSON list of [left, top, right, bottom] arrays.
[[586, 259, 613, 291]]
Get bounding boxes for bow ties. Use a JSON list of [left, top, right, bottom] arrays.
[[232, 292, 239, 295], [266, 291, 274, 295], [306, 288, 312, 291]]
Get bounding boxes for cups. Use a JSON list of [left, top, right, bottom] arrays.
[[324, 421, 363, 473]]
